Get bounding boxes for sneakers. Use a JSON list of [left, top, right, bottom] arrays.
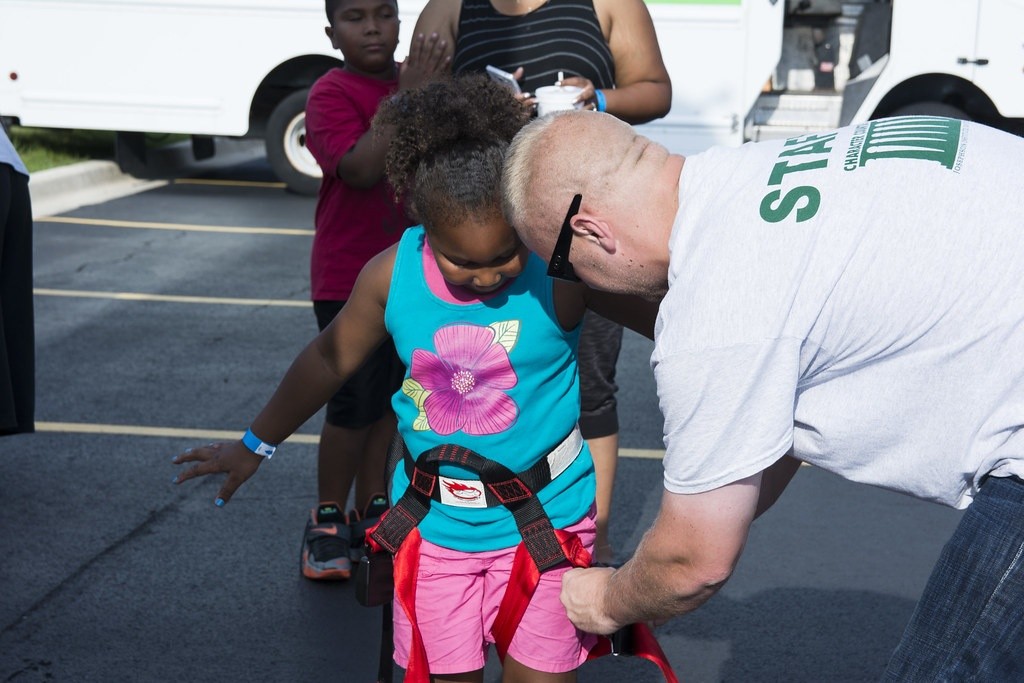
[[349, 493, 390, 559], [302, 502, 351, 580]]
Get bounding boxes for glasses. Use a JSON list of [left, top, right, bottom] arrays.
[[547, 194, 582, 283]]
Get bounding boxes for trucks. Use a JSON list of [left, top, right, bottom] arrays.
[[0, 0, 1024, 203]]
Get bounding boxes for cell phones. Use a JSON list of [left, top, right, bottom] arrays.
[[485, 64, 521, 95]]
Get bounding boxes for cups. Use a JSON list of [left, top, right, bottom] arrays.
[[535, 84, 587, 117]]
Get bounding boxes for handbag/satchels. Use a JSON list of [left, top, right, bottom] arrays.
[[355, 550, 394, 607]]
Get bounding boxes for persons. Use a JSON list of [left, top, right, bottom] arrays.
[[171, 0, 673, 683], [503, 110, 1023, 682]]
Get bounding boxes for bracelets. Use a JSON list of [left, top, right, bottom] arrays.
[[241, 426, 277, 460], [594, 88, 606, 113]]
